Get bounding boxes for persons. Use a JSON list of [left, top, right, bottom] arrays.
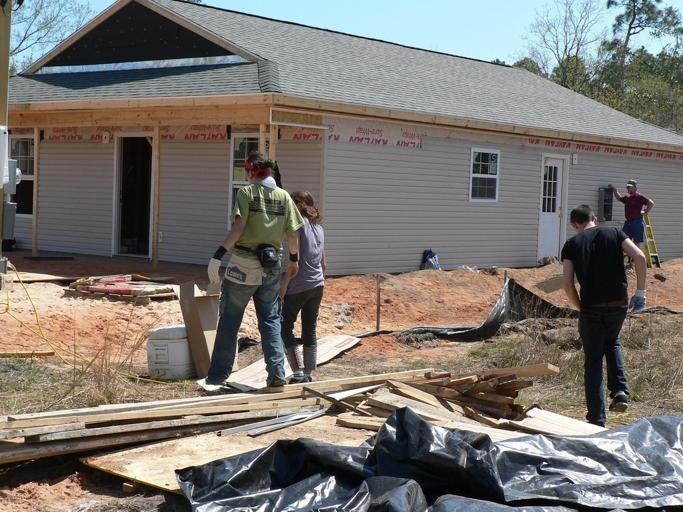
[[608, 177, 654, 267], [279, 189, 327, 384], [194, 147, 304, 389], [560, 203, 648, 430]]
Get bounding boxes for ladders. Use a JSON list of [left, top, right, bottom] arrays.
[[641, 208, 662, 268]]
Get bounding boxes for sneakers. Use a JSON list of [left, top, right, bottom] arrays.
[[267, 378, 287, 389], [205, 377, 226, 386], [288, 376, 313, 385], [609, 395, 629, 412]]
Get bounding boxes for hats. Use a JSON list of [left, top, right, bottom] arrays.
[[626, 180, 637, 187]]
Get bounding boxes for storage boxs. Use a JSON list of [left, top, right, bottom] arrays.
[[180, 280, 238, 378], [147, 323, 197, 381]]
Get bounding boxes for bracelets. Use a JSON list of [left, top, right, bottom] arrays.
[[287, 251, 301, 262], [213, 245, 228, 261]]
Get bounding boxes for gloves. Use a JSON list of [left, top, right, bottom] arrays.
[[207, 257, 223, 285], [629, 289, 647, 313]]
